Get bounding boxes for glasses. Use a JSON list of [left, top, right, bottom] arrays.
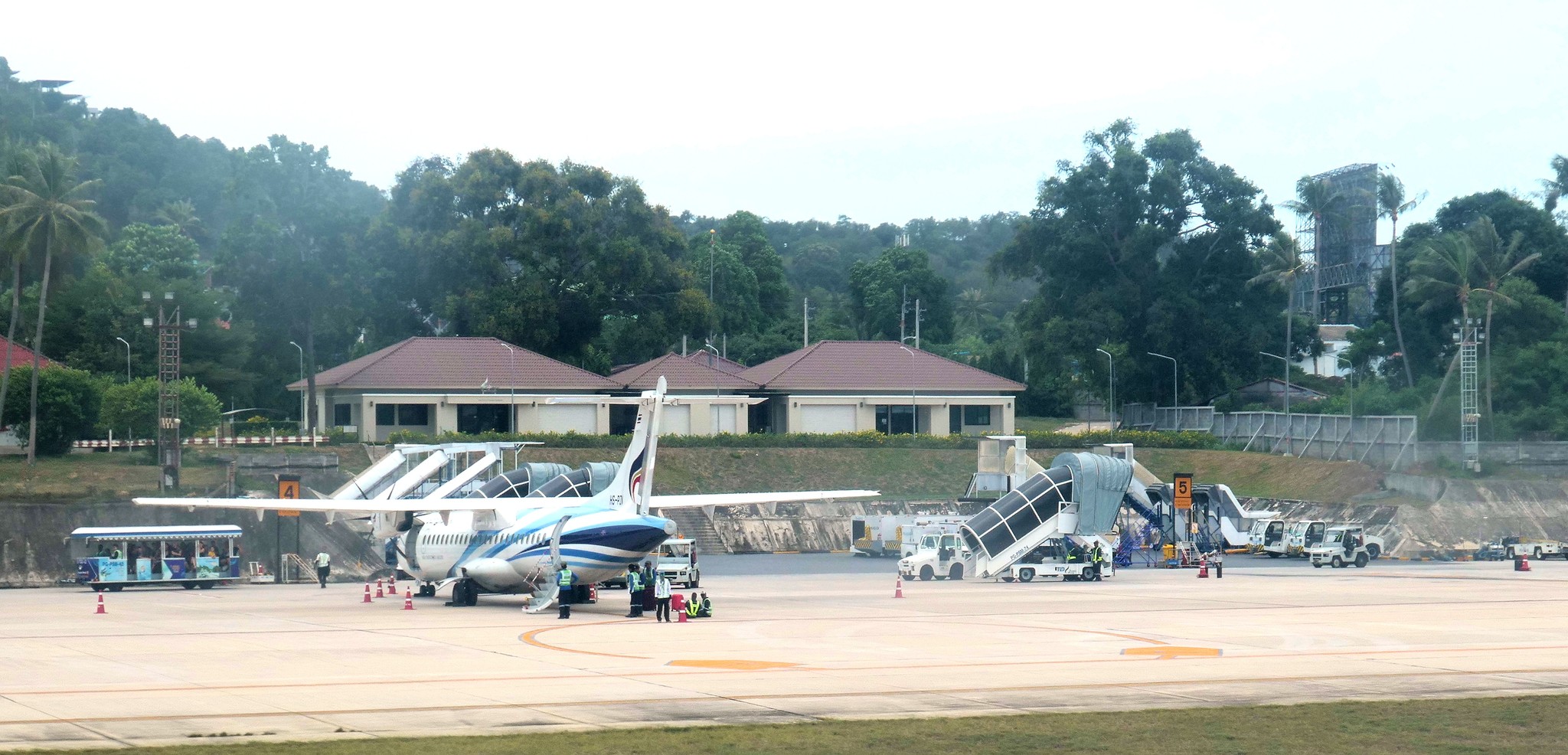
[[628, 568, 630, 569]]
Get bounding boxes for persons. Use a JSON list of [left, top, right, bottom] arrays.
[[95, 540, 243, 572], [643, 561, 656, 611], [625, 564, 646, 617], [696, 590, 712, 617], [655, 571, 672, 623], [556, 561, 579, 619], [314, 546, 331, 588], [685, 593, 702, 618], [1065, 540, 1102, 582]]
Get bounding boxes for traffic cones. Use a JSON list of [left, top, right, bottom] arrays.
[[399, 586, 417, 610], [1198, 557, 1208, 577], [1516, 550, 1532, 571], [588, 583, 596, 600], [95, 589, 108, 614], [359, 581, 374, 603], [372, 575, 386, 598], [386, 573, 398, 594], [673, 600, 693, 623], [258, 562, 264, 575], [891, 576, 907, 598]]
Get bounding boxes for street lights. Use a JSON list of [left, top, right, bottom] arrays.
[[1148, 352, 1177, 431], [1325, 354, 1354, 461], [900, 346, 916, 439], [745, 353, 756, 370], [1260, 351, 1291, 454], [705, 344, 720, 436], [500, 343, 514, 433], [1096, 348, 1114, 444], [290, 341, 304, 447], [116, 337, 130, 376]]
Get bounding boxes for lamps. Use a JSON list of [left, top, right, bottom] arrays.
[[794, 402, 796, 407], [602, 403, 605, 407], [370, 401, 373, 407], [944, 403, 946, 408], [532, 402, 535, 407], [442, 402, 445, 407], [741, 403, 743, 408], [860, 402, 863, 408], [1008, 402, 1011, 408]]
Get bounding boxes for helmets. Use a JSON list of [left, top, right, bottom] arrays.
[[628, 564, 636, 569], [645, 561, 652, 566], [634, 564, 640, 569]]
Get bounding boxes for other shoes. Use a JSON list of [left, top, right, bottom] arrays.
[[658, 620, 662, 622], [558, 616, 564, 618], [666, 620, 671, 622], [638, 612, 643, 616], [564, 615, 569, 618], [626, 614, 632, 617]]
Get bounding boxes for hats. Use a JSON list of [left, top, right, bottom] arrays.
[[1094, 541, 1099, 544], [561, 561, 567, 566], [701, 591, 706, 594], [1074, 544, 1080, 548], [1084, 545, 1090, 549], [660, 572, 665, 576]]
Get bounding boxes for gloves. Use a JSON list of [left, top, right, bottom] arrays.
[[1095, 560, 1098, 564], [655, 597, 658, 600], [670, 595, 672, 599]]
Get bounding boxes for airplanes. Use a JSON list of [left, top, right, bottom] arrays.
[[129, 373, 885, 614]]
